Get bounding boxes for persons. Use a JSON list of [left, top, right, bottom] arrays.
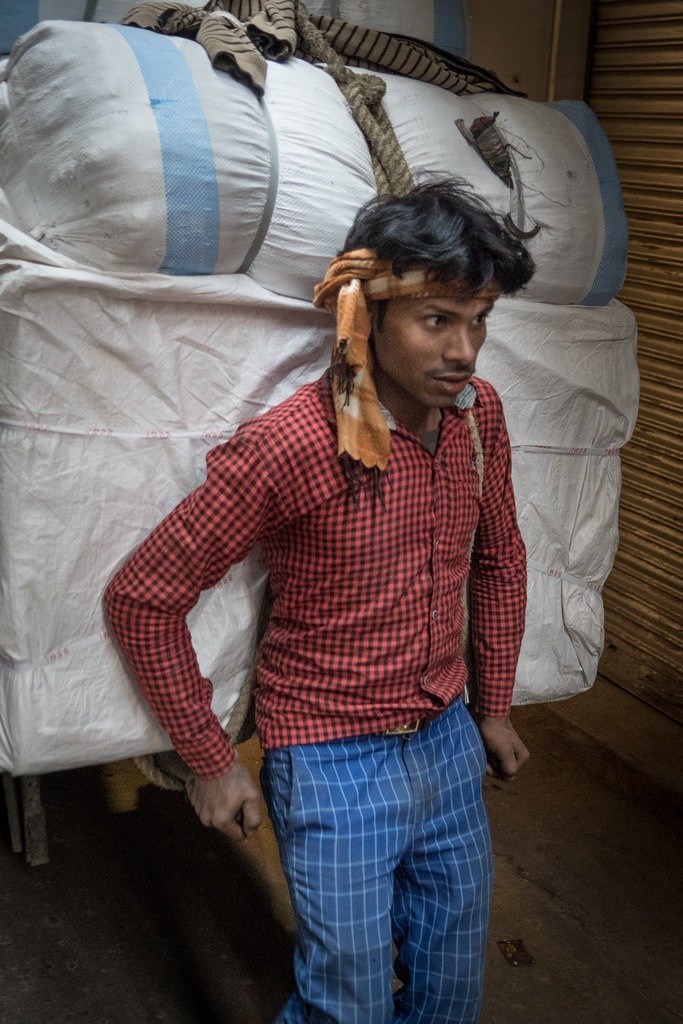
[[102, 171, 535, 1024]]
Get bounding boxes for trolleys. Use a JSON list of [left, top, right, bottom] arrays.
[[3, 732, 277, 871]]
